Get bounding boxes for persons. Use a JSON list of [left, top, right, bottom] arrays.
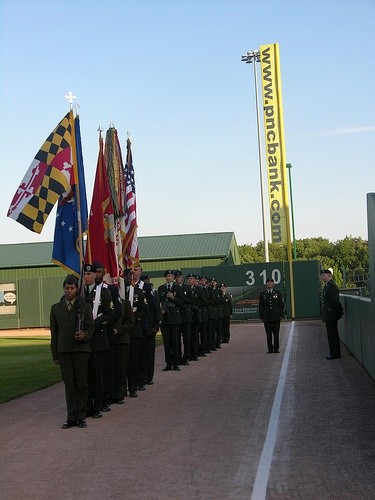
[[320, 270, 344, 360], [157, 269, 232, 371], [49, 259, 162, 429], [259, 278, 285, 354]]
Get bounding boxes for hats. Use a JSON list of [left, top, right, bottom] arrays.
[[164, 270, 226, 288], [92, 261, 104, 269], [64, 274, 77, 285], [140, 274, 151, 281], [266, 278, 274, 283], [84, 264, 96, 273], [320, 270, 331, 274], [124, 269, 133, 277]]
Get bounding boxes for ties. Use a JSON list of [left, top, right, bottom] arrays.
[[68, 303, 71, 310], [86, 286, 90, 297], [128, 289, 130, 299], [269, 290, 271, 297], [168, 284, 170, 292]]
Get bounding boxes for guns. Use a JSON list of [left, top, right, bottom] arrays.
[[75, 274, 87, 336], [161, 288, 169, 313]]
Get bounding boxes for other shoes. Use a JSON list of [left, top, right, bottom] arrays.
[[62, 419, 77, 429], [105, 399, 124, 405], [129, 390, 138, 398], [267, 351, 274, 354], [162, 339, 230, 371], [87, 409, 103, 418], [146, 380, 154, 385], [326, 355, 341, 359], [77, 419, 88, 428], [139, 383, 145, 391], [274, 349, 281, 353], [94, 402, 110, 412]]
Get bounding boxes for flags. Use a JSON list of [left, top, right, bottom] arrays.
[[85, 137, 140, 283], [8, 112, 75, 234], [50, 114, 89, 279]]
